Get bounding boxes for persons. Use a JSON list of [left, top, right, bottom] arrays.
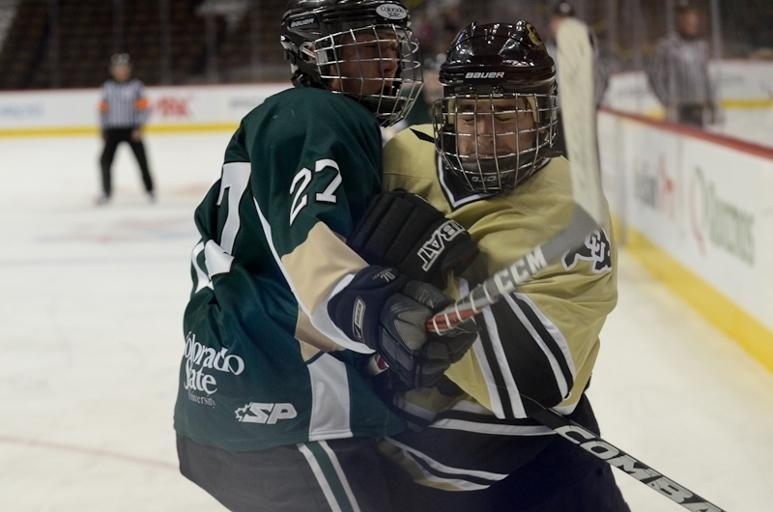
[[88, 50, 158, 205], [640, 1, 720, 127], [369, 17, 632, 512], [535, 2, 613, 157], [166, 0, 481, 512]]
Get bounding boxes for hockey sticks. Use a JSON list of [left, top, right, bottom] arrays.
[[370, 19, 605, 377]]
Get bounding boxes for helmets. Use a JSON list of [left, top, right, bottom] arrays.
[[279, 0, 424, 62], [438, 19, 557, 125]]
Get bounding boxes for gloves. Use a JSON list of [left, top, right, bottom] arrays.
[[327, 187, 478, 390]]
